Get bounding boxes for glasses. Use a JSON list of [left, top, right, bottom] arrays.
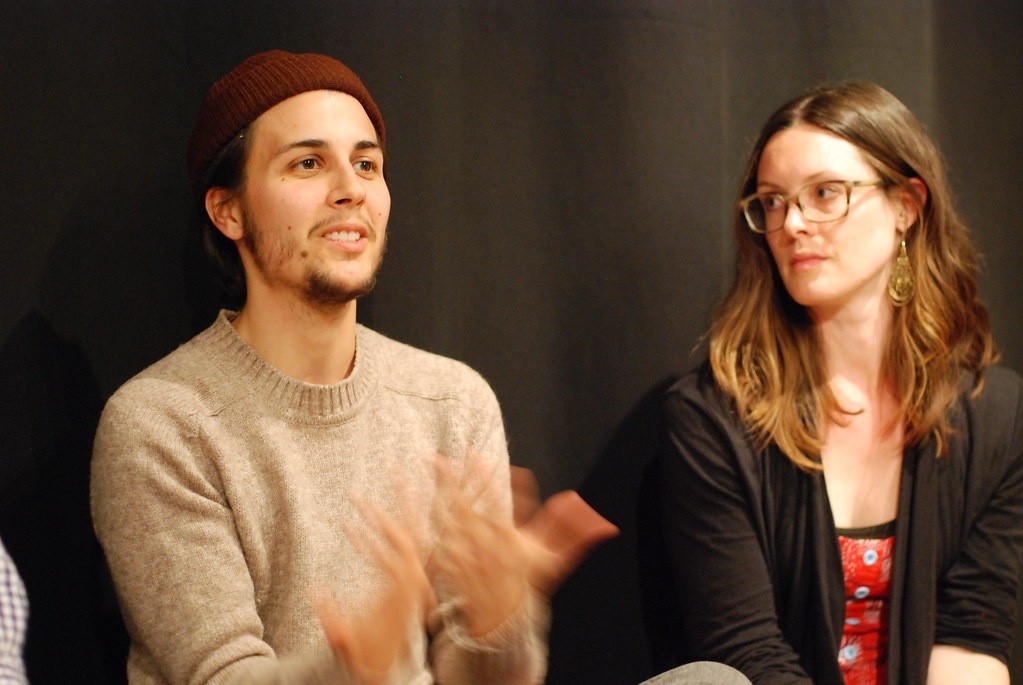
[[739, 178, 896, 234]]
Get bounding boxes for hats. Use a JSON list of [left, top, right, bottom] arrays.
[[187, 49, 386, 192]]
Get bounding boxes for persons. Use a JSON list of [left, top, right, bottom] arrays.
[[0, 538, 31, 685], [84, 48, 752, 685], [663, 81, 1023, 685]]
